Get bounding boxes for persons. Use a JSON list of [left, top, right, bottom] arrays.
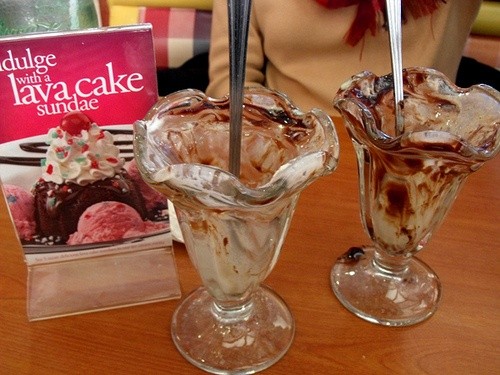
[[210, 0, 486, 120]]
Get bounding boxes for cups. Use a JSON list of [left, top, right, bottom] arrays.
[[328, 66, 500, 327], [131, 86, 339, 375]]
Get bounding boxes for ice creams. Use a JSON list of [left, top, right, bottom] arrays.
[[1, 110, 168, 245]]
